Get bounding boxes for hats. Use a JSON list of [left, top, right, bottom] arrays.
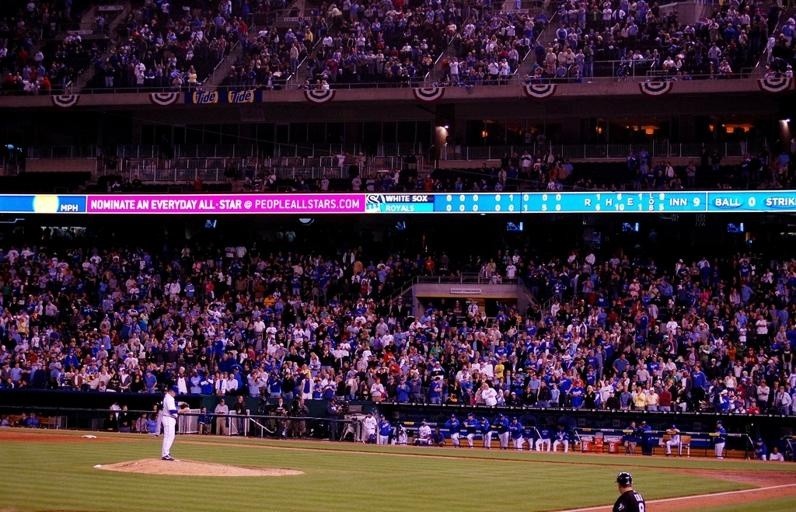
[[169, 385, 178, 393], [451, 412, 517, 421]]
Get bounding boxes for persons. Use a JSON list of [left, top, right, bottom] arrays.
[[612, 471, 646, 511], [0, 0, 794, 461]]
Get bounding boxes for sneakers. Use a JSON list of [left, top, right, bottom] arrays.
[[161, 455, 173, 460]]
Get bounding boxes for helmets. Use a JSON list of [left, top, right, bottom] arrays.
[[614, 473, 632, 485]]
